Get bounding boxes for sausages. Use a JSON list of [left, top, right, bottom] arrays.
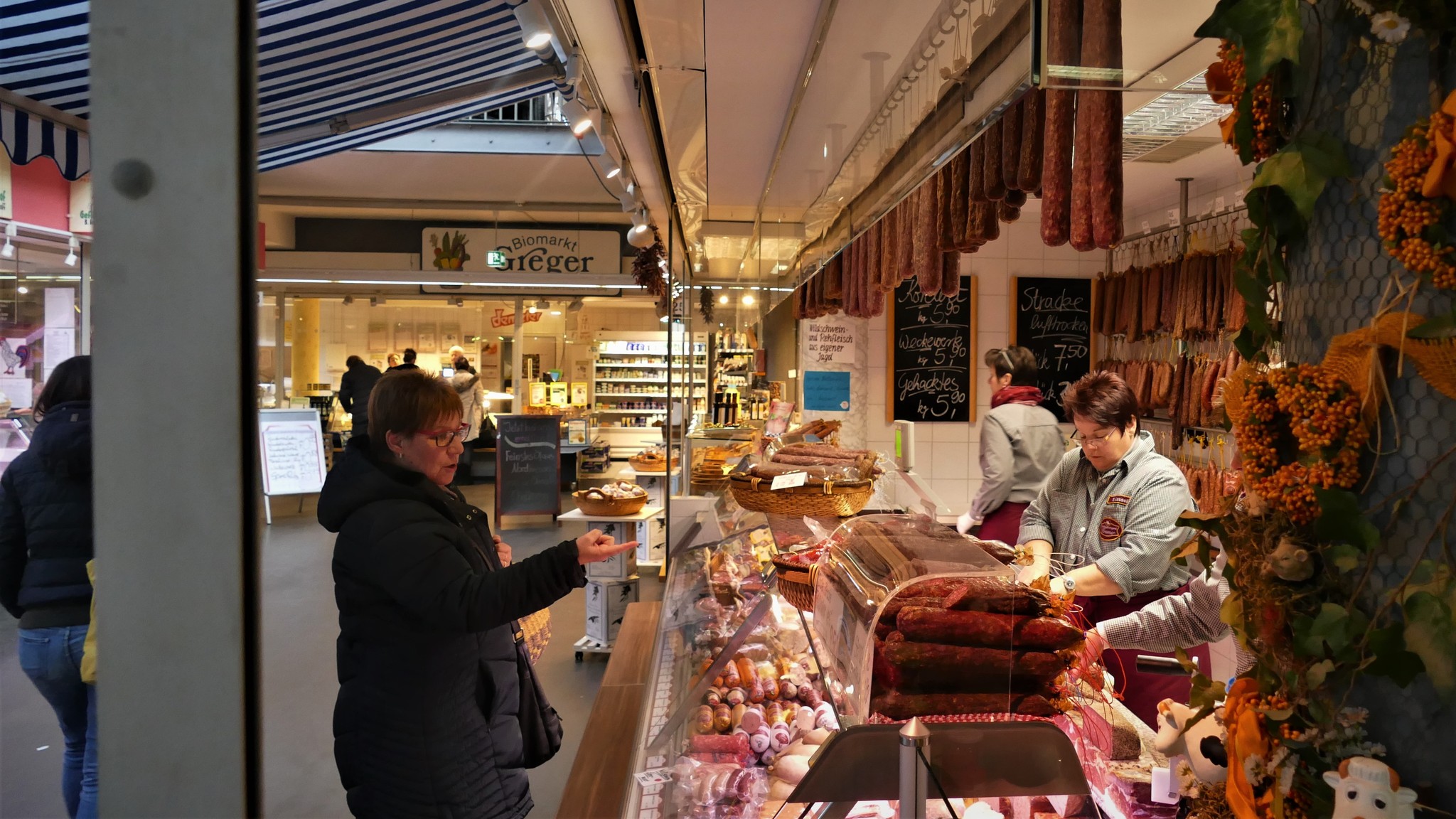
[[1094, 350, 1280, 451], [1175, 460, 1228, 514], [749, 444, 883, 482], [1099, 246, 1245, 342], [653, 628, 842, 819], [790, 419, 840, 439], [861, 576, 1086, 718], [792, 0, 1122, 318]]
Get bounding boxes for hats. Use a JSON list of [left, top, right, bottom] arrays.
[[449, 346, 464, 355]]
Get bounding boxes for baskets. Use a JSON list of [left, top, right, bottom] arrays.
[[728, 477, 876, 517], [772, 547, 842, 613]]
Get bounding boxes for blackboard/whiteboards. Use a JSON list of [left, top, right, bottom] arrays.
[[494, 413, 562, 516], [1008, 275, 1099, 423], [259, 408, 328, 496], [885, 274, 977, 425]]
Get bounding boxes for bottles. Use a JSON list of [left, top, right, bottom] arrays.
[[327, 396, 331, 416], [750, 397, 757, 420], [317, 399, 321, 416], [657, 370, 668, 379], [684, 371, 689, 382], [672, 386, 682, 397], [712, 392, 725, 425], [757, 396, 764, 419], [684, 386, 689, 397], [693, 371, 701, 380], [715, 384, 738, 403], [725, 393, 732, 424], [732, 393, 738, 424], [312, 399, 317, 408], [697, 398, 705, 411], [322, 397, 327, 416], [671, 371, 681, 380], [695, 386, 705, 397], [648, 384, 667, 393]]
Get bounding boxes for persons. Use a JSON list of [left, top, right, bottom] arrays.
[[340, 347, 490, 485], [957, 346, 1256, 733], [0, 355, 100, 819], [317, 367, 637, 819]]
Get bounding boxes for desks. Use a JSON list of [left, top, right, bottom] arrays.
[[617, 465, 682, 567], [556, 504, 665, 663]]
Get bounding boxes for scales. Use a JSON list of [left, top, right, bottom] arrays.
[[893, 419, 952, 522]]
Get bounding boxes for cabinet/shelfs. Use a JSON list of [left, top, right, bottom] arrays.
[[589, 330, 710, 461]]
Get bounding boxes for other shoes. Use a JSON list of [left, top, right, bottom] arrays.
[[459, 476, 474, 483]]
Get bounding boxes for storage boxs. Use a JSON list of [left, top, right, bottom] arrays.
[[579, 435, 612, 474]]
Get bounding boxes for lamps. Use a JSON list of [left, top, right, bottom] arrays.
[[342, 295, 353, 306], [629, 187, 647, 233], [370, 296, 376, 306], [618, 159, 637, 214], [597, 112, 621, 179], [513, 0, 552, 48], [534, 299, 551, 310], [1, 223, 17, 259], [65, 236, 79, 267], [456, 298, 464, 307], [567, 296, 584, 316], [561, 46, 593, 134], [657, 254, 666, 268]]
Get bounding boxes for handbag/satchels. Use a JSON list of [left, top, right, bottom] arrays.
[[480, 415, 498, 443], [513, 637, 563, 769]]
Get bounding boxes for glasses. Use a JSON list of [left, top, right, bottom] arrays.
[[1069, 427, 1115, 446], [998, 348, 1014, 369], [414, 423, 472, 448]]
